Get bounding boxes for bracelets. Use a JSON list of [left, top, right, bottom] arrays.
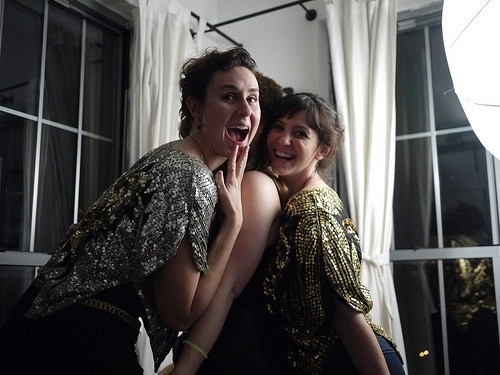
[[183, 340, 208, 360]]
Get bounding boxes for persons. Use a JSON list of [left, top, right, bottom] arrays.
[[246, 87, 406, 375], [168, 70, 294, 375], [440, 233, 499, 375], [1, 45, 260, 375]]
